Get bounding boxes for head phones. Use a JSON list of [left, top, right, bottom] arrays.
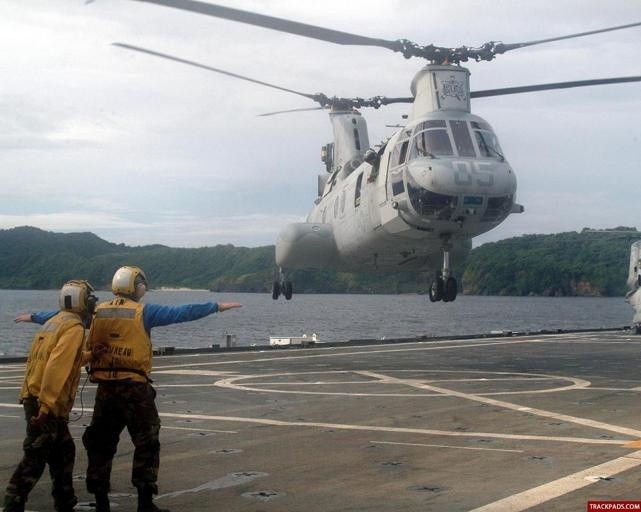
[[135, 282, 145, 298], [87, 295, 98, 311]]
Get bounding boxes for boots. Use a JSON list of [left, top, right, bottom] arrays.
[[137, 490, 169, 511], [96, 493, 111, 512]]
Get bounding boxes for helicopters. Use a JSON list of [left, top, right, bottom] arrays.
[[86, 0, 641, 302]]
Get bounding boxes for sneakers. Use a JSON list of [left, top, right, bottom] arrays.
[[54, 495, 77, 510], [2, 504, 25, 511]]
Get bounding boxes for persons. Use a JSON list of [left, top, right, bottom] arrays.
[[0, 280, 107, 512], [14, 266, 242, 511]]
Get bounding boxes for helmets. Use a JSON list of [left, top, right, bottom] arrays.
[[59, 280, 98, 314], [112, 265, 149, 302]]
